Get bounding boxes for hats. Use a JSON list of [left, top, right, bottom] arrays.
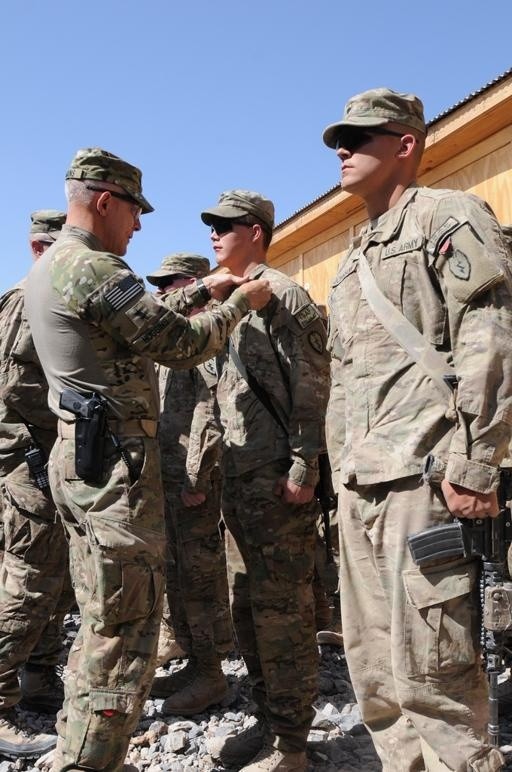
[[64, 146, 155, 215], [29, 211, 68, 242], [145, 253, 211, 287], [322, 87, 427, 149], [201, 190, 275, 229]]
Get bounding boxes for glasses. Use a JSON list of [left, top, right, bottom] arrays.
[[336, 133, 420, 150], [211, 222, 253, 236], [87, 186, 143, 220], [158, 277, 190, 289]]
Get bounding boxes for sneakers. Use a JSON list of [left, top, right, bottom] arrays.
[[219, 720, 277, 768], [238, 744, 308, 772], [161, 668, 229, 717], [18, 670, 65, 711], [150, 662, 194, 699], [315, 617, 347, 648], [0, 706, 58, 757]]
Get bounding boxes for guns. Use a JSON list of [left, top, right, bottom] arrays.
[[405, 374, 512, 751], [302, 446, 338, 567], [58, 389, 105, 483]]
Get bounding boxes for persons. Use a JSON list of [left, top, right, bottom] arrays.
[[321, 86, 510, 772], [145, 248, 236, 718], [20, 147, 273, 769], [276, 297, 345, 648], [198, 187, 338, 772], [0, 207, 67, 756]]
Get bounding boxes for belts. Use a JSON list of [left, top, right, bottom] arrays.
[[56, 418, 161, 440]]
